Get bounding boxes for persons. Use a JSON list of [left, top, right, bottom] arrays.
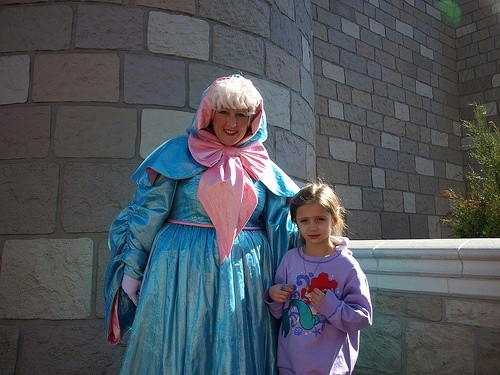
[[104, 75, 301, 375], [263, 183, 373, 375]]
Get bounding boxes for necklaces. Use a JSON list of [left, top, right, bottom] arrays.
[[303, 246, 325, 277]]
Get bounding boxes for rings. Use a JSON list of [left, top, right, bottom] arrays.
[[280, 286, 287, 291]]
